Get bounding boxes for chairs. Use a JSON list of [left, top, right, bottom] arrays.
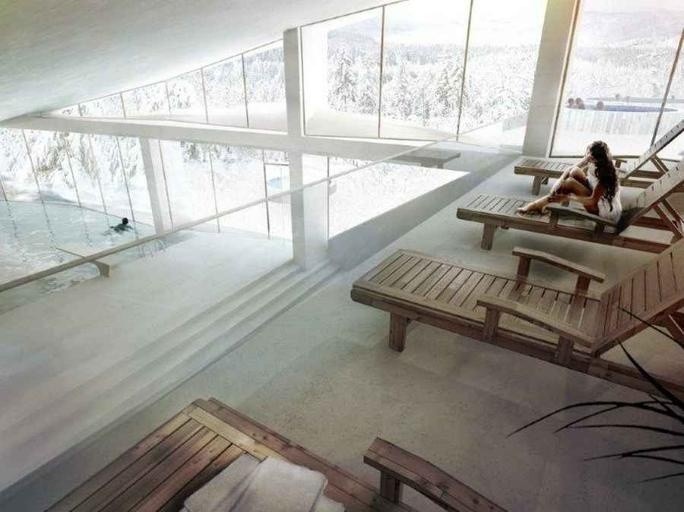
[[45, 397, 510, 511], [352, 247, 659, 395], [456, 159, 684, 255], [515, 120, 684, 196]]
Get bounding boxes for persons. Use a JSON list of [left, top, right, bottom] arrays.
[[567, 99, 574, 108], [112, 218, 131, 233], [517, 142, 621, 224], [572, 98, 581, 108]]
